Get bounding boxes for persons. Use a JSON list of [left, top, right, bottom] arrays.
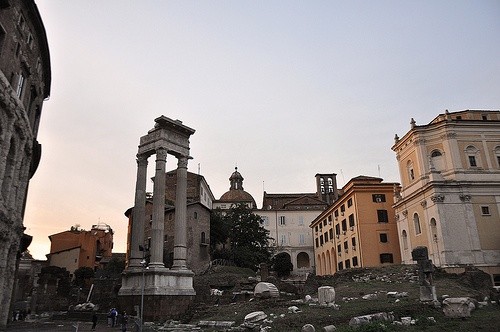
[[13, 310, 17, 322], [91, 311, 97, 331], [122, 312, 128, 332]]
